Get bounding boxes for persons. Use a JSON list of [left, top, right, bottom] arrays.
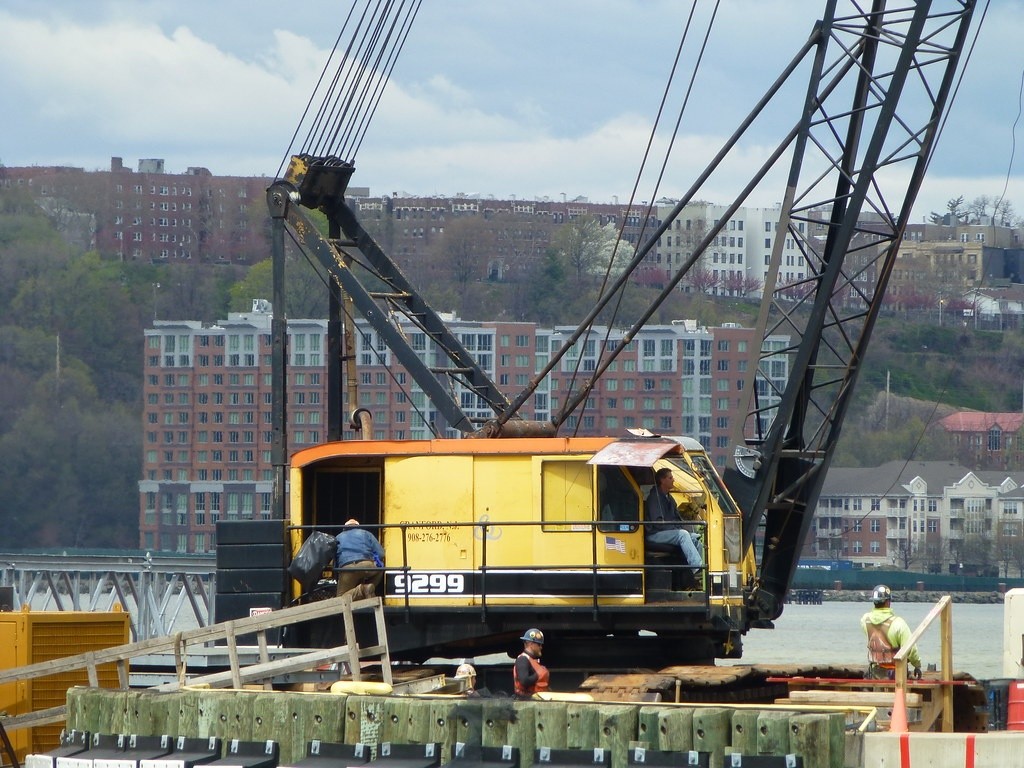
[[334, 519, 386, 602], [858, 583, 922, 681], [644, 468, 706, 589], [453, 664, 477, 692], [513, 628, 550, 699]]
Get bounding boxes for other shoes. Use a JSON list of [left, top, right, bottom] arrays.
[[363, 583, 376, 600], [692, 566, 703, 575], [376, 584, 391, 600]]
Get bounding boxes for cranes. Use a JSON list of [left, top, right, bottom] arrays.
[[214, 0, 993, 675]]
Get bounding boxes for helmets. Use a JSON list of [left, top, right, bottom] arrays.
[[343, 519, 359, 529], [454, 664, 476, 679], [871, 584, 893, 604], [520, 628, 544, 644]]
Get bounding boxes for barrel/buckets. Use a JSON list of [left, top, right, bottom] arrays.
[[1007, 681, 1024, 731]]
